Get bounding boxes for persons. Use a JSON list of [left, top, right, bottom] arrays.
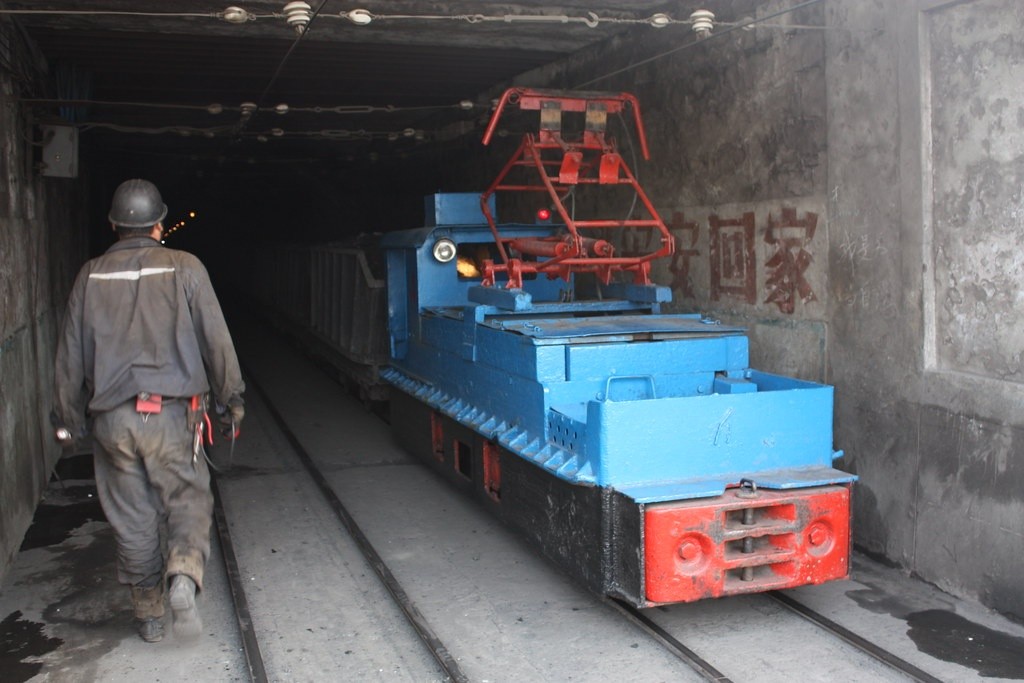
[[49, 178, 247, 643]]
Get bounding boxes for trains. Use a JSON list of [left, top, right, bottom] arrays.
[[285, 85, 859, 609]]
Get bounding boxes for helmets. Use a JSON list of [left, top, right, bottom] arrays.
[[108, 178, 169, 228]]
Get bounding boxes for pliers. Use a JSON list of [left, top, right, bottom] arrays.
[[196, 399, 214, 446]]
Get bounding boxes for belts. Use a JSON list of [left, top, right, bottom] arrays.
[[162, 395, 183, 404]]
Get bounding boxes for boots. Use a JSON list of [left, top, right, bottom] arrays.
[[132, 582, 164, 645], [169, 573, 203, 641]]
